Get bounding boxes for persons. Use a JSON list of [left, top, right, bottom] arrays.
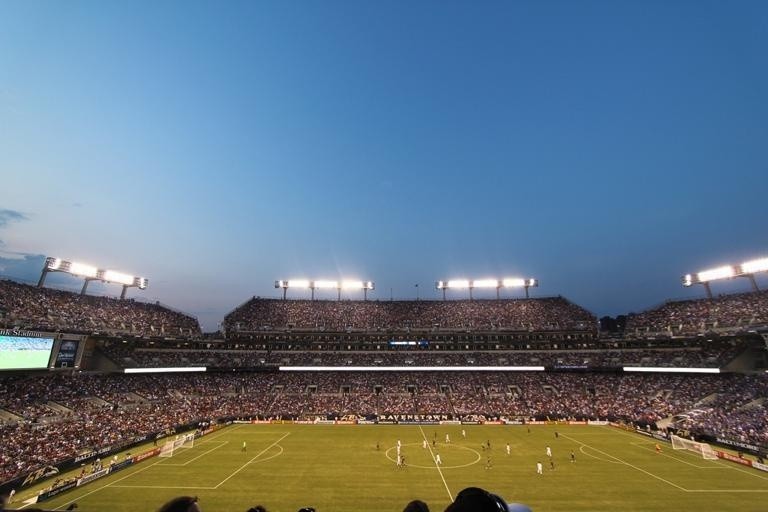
[[443, 487, 509, 511], [376, 425, 578, 480], [241, 440, 247, 457], [616, 289, 767, 461], [157, 495, 201, 512], [223, 297, 616, 424], [0, 279, 225, 439], [0, 439, 159, 496], [8, 488, 16, 503]]
